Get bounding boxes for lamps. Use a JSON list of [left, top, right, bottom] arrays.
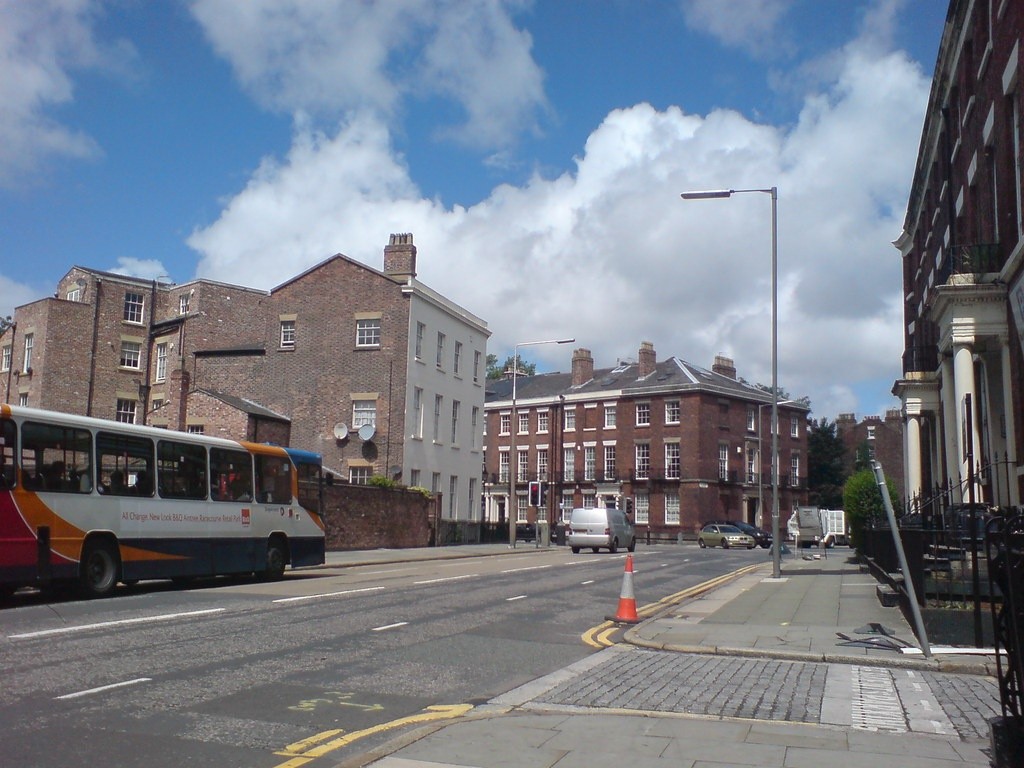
[[13, 368, 20, 376], [27, 367, 32, 374]]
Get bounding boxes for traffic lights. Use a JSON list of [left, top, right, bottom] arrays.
[[541, 484, 547, 507], [528, 481, 540, 506]]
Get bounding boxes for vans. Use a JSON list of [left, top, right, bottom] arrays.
[[568, 506, 636, 554]]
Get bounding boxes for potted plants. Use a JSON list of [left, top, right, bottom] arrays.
[[956, 247, 975, 274]]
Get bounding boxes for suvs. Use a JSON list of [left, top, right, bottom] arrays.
[[700, 519, 774, 549]]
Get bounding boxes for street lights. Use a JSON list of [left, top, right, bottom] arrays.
[[507, 338, 575, 550], [679, 186, 783, 578], [757, 399, 794, 529]]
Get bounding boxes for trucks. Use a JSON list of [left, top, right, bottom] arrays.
[[787, 507, 853, 549]]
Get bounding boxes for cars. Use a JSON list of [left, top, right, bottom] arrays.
[[696, 524, 755, 550]]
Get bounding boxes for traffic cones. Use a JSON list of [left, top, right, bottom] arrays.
[[615, 554, 640, 622]]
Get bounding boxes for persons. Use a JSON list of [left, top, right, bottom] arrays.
[[230, 479, 252, 503], [44, 460, 223, 503]]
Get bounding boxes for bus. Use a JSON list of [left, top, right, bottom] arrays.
[[0, 401, 326, 602]]
